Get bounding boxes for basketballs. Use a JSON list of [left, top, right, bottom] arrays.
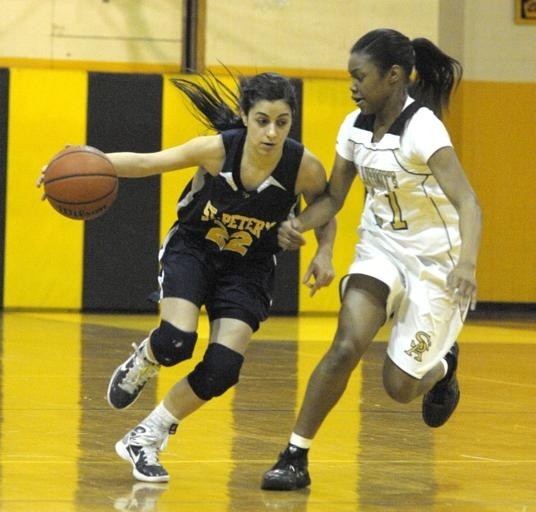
[[44, 145, 118, 220]]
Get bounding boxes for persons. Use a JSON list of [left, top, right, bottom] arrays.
[[261, 29, 482, 490], [36, 58, 336, 483]]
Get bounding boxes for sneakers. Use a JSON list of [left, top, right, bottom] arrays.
[[107, 337, 161, 410], [262, 450, 310, 490], [115, 424, 170, 482], [422, 342, 460, 427]]
